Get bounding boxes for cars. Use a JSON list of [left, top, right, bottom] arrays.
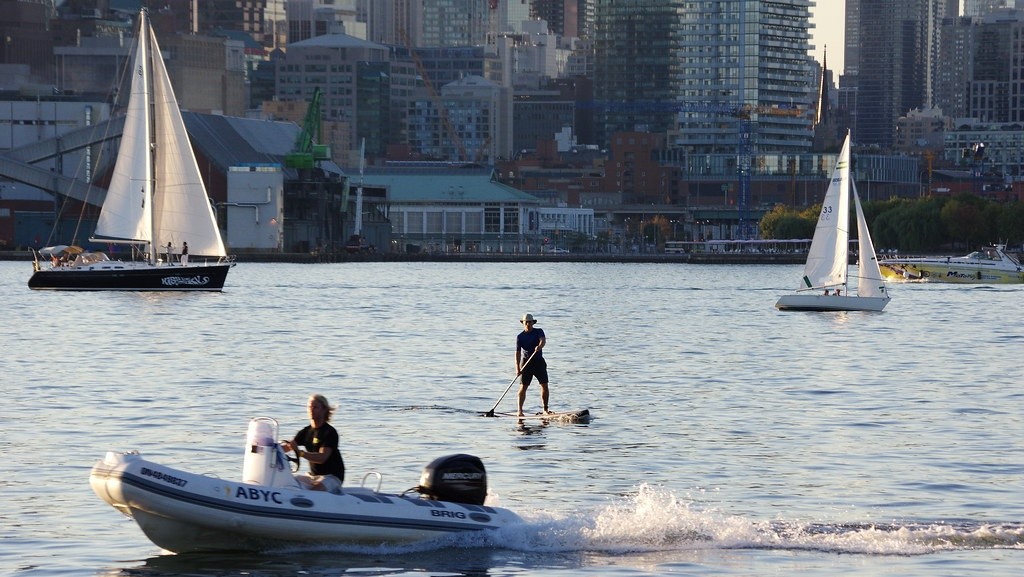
[[343, 240, 375, 253], [547, 248, 569, 255], [984, 182, 1014, 192]]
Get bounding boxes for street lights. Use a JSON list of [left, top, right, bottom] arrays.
[[962, 141, 985, 193]]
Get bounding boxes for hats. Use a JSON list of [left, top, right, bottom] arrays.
[[520, 314, 537, 324]]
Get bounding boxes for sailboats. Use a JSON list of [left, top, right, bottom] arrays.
[[28, 6, 237, 293], [774, 128, 892, 311]]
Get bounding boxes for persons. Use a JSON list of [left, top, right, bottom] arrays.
[[160, 242, 176, 266], [51, 253, 75, 269], [824, 290, 830, 296], [515, 313, 552, 417], [279, 394, 348, 492], [833, 289, 841, 296], [178, 241, 188, 267]]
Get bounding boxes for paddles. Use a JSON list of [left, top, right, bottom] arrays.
[[484, 350, 537, 418]]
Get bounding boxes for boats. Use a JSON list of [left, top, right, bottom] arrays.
[[88, 418, 526, 554], [857, 245, 1024, 284]]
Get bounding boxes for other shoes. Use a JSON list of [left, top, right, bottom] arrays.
[[518, 413, 524, 417], [543, 411, 551, 414]]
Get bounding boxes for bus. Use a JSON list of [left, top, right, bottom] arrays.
[[664, 241, 705, 254]]
